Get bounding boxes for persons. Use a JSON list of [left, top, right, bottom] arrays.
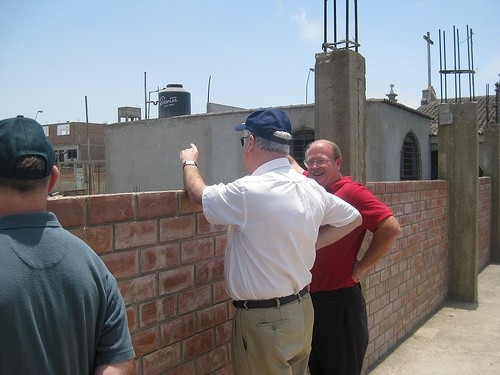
[[289, 140, 401, 375], [0, 115, 137, 375], [179, 108, 362, 375]]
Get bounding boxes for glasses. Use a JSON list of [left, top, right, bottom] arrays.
[[304, 157, 338, 169], [241, 135, 260, 147]]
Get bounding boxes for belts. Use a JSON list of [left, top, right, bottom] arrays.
[[233, 285, 308, 309]]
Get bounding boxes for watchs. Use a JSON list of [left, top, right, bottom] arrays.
[[182, 160, 198, 169]]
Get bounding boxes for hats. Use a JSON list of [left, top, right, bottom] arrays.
[[235, 107, 292, 145], [0, 115, 54, 179]]
